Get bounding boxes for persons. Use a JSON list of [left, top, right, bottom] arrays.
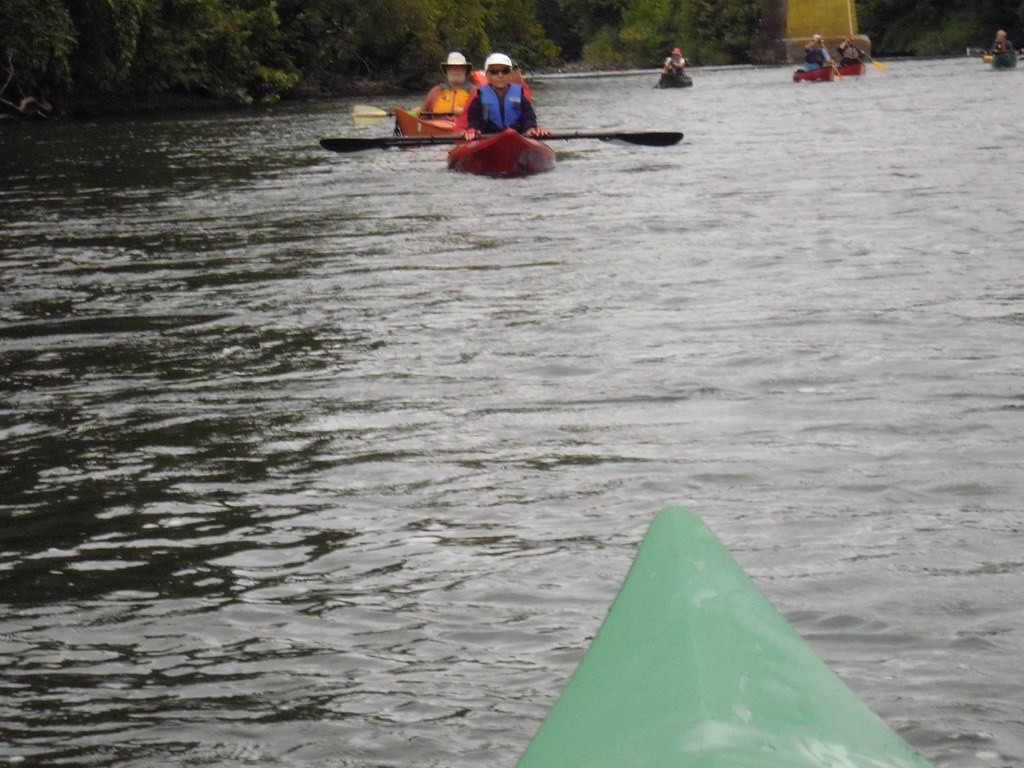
[[990, 30, 1014, 56], [461, 53, 548, 143], [663, 49, 685, 79], [837, 35, 865, 66], [412, 52, 470, 121], [804, 34, 835, 74]]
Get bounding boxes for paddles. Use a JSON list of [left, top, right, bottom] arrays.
[[821, 40, 844, 81], [315, 129, 684, 155], [847, 40, 886, 73], [347, 103, 456, 128]]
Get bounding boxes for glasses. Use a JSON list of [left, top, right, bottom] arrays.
[[488, 67, 510, 75]]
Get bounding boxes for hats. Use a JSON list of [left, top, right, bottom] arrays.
[[811, 35, 823, 42], [485, 53, 512, 72], [441, 52, 472, 76], [672, 48, 681, 55]]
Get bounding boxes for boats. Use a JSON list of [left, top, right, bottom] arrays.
[[990, 50, 1019, 70], [390, 106, 462, 140], [792, 65, 837, 84], [656, 66, 694, 89], [836, 61, 867, 77], [453, 129, 556, 177]]
